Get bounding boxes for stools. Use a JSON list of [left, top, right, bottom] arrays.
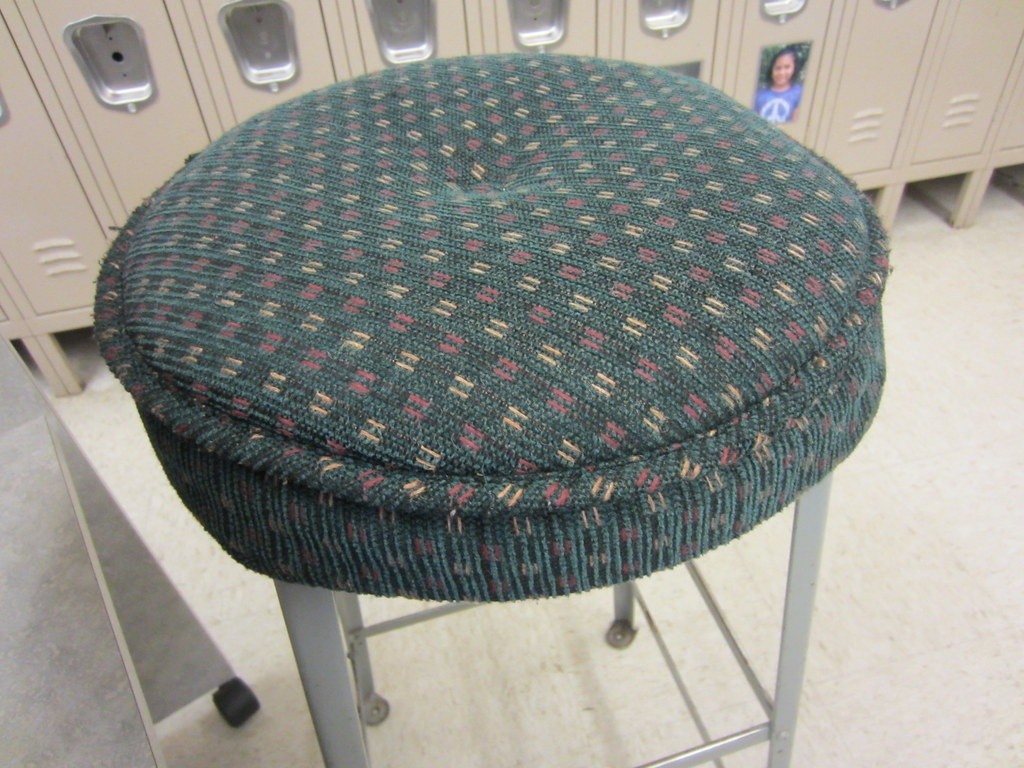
[[95, 53, 891, 768]]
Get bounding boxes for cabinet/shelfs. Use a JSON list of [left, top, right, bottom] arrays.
[[0, 0, 1024, 398]]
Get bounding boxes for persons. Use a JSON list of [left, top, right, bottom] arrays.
[[756, 49, 802, 120]]
